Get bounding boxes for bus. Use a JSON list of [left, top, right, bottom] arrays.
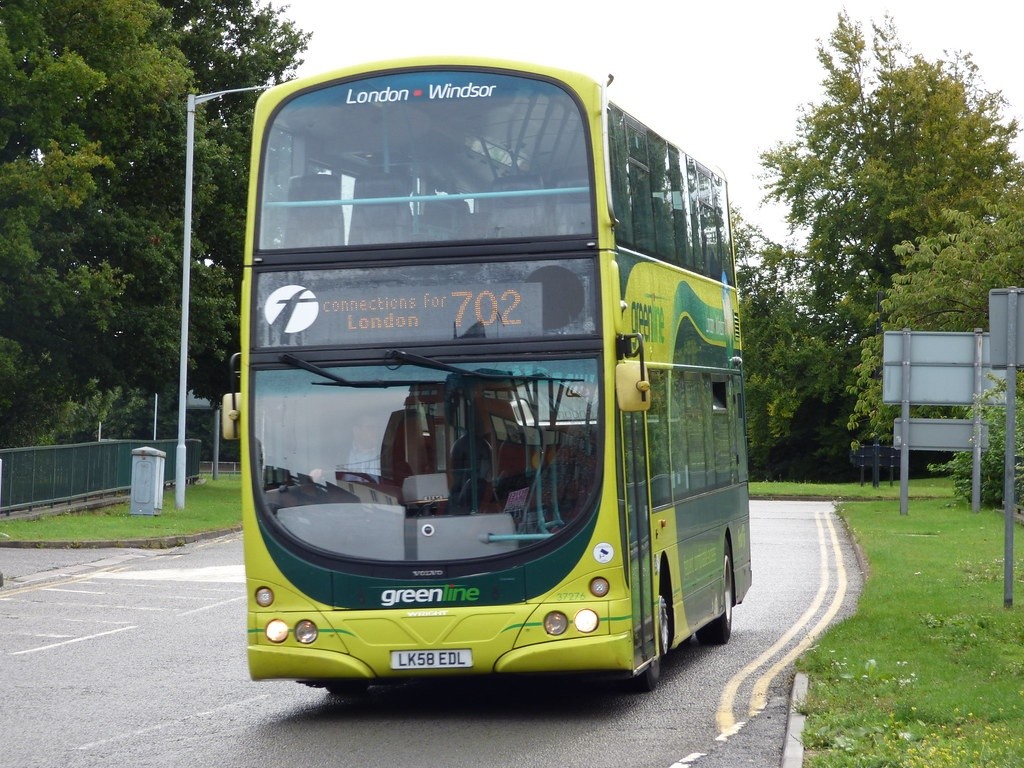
[[220, 55, 752, 696]]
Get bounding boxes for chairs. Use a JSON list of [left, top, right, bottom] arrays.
[[484, 175, 550, 237], [285, 174, 344, 248], [422, 200, 473, 234], [349, 173, 413, 245], [497, 440, 527, 513], [551, 178, 592, 235], [439, 431, 494, 514]]
